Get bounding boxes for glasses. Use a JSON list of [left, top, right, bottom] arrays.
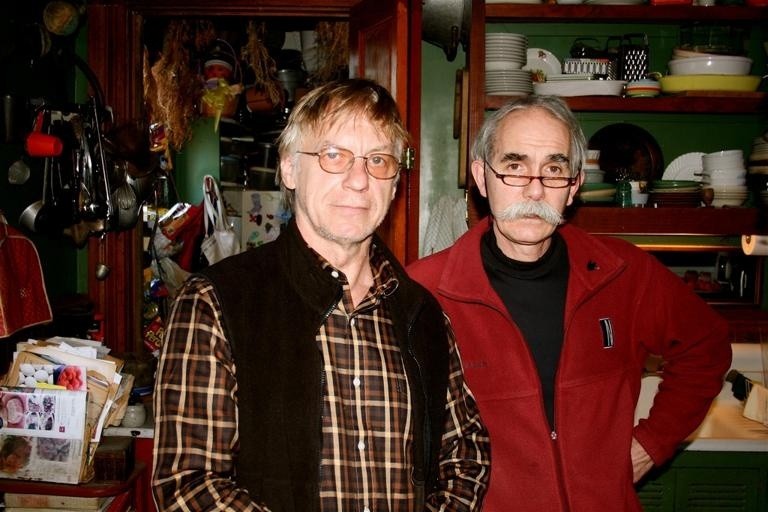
[[485, 160, 583, 188], [296, 149, 404, 179]]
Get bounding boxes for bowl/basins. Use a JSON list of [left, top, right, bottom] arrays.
[[650, 149, 748, 208], [661, 49, 762, 94], [576, 151, 616, 204]]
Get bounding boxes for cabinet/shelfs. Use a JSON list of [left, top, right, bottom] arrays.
[[468, 0, 766, 510], [88, 0, 422, 475], [0, 462, 144, 512]]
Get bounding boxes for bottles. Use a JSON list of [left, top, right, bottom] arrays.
[[616, 181, 631, 208]]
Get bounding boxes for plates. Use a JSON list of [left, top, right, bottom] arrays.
[[484, 32, 660, 97], [587, 123, 664, 193]]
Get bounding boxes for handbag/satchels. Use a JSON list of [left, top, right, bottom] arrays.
[[201, 226, 240, 266]]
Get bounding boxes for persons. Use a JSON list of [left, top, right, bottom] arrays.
[[150, 75, 492, 511], [401, 92, 732, 511]]
